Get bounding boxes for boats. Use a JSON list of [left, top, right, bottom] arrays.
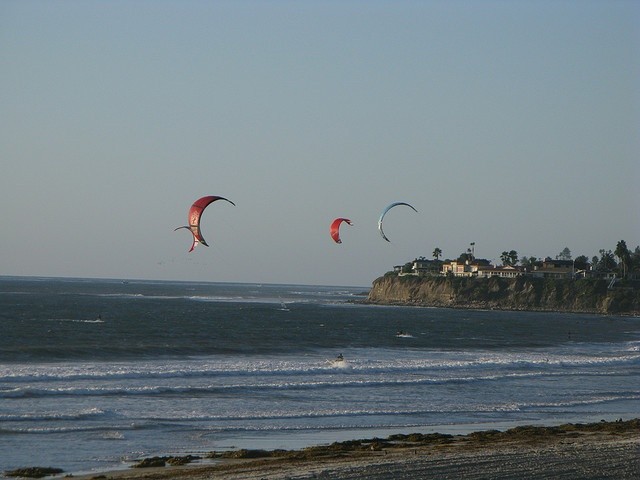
[[394, 329, 414, 337], [336, 353, 344, 363]]
